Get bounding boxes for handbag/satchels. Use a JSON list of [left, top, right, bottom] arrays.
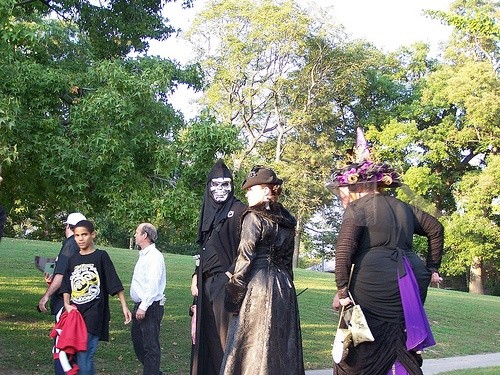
[[333, 303, 352, 363], [342, 291, 375, 345]]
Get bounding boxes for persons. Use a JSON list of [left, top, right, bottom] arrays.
[[40, 213, 88, 323], [220, 166, 305, 375], [326, 164, 444, 375], [50, 220, 132, 375], [189, 158, 247, 375], [130, 223, 167, 375]]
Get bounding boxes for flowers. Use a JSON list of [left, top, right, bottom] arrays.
[[331, 161, 400, 184]]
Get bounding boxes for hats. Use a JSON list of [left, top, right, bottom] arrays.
[[242, 167, 283, 188], [63, 213, 86, 225]]
